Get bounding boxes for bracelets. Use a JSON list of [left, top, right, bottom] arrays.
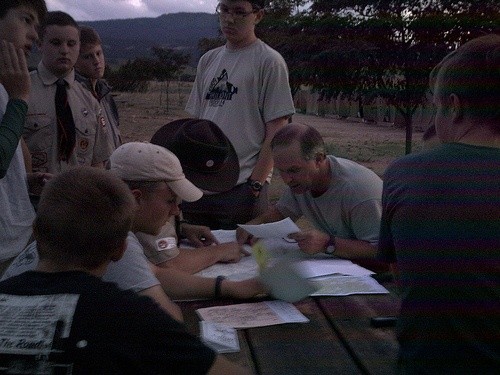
[[216, 274, 226, 296], [180, 220, 190, 224], [248, 233, 253, 242]]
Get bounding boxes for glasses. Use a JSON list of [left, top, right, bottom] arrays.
[[216, 3, 256, 18]]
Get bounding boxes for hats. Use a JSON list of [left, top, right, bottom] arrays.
[[110, 142, 203, 202], [152, 118, 240, 192]]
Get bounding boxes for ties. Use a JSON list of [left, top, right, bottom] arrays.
[[54, 83, 77, 164]]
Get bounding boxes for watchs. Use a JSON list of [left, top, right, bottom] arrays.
[[247, 178, 264, 192], [324, 236, 337, 254]]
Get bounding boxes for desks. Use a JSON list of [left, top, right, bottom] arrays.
[[176, 297, 401, 375]]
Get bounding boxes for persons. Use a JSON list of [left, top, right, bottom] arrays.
[[375, 33, 500, 375], [133, 118, 252, 274], [0, 141, 204, 322], [0, 166, 252, 375], [421, 128, 441, 152], [74, 25, 122, 149], [30, 11, 113, 211], [184, 0, 295, 230], [0, 0, 47, 278], [238, 122, 383, 259]]
